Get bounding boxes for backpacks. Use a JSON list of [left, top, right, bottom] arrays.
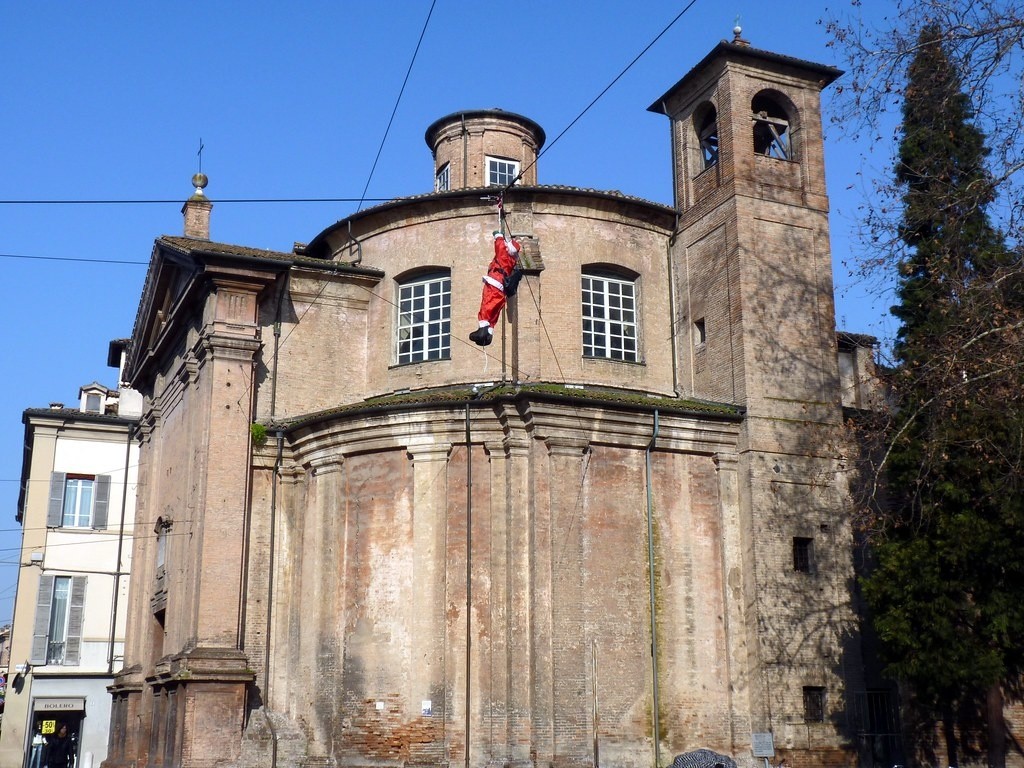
[[493, 255, 524, 297]]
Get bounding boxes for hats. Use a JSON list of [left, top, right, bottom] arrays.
[[507, 240, 520, 256]]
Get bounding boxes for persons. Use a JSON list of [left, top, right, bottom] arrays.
[[469, 230, 520, 347], [29, 720, 74, 768]]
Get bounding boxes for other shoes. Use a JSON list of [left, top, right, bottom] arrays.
[[468, 321, 495, 346]]
